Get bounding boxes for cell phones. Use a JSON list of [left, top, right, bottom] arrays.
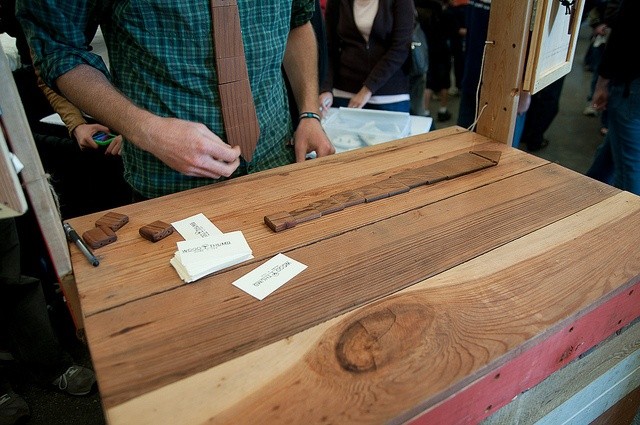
[[92, 130, 117, 146]]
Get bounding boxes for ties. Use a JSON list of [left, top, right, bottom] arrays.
[[211, 0, 260, 164]]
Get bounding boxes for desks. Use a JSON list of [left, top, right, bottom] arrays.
[[62, 124, 639, 425]]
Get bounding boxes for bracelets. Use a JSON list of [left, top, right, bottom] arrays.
[[298, 112, 320, 122]]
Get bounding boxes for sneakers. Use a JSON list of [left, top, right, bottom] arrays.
[[1, 389, 30, 423], [583, 100, 598, 116], [438, 108, 451, 122], [52, 363, 96, 395]]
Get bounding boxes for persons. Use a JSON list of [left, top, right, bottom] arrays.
[[415, 1, 453, 123], [318, 0, 413, 118], [583, 1, 639, 196], [517, 76, 567, 152], [26, 0, 337, 203], [456, 4, 493, 130], [25, 24, 126, 157], [0, 210, 99, 424]]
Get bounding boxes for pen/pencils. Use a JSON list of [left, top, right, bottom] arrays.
[[63, 222, 98, 266]]
